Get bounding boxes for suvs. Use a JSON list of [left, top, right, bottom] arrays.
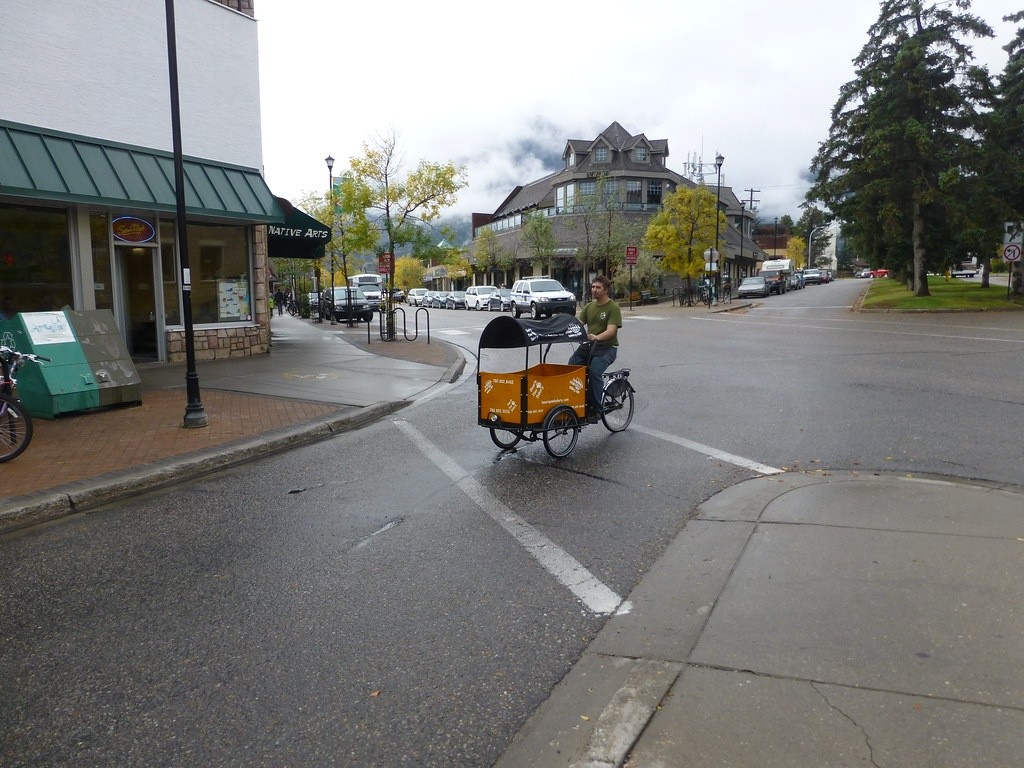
[[509, 275, 577, 320]]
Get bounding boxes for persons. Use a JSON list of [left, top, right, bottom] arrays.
[[268, 287, 291, 317], [567, 276, 623, 423]]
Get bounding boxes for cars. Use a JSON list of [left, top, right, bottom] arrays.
[[803, 269, 822, 286], [737, 277, 770, 298], [855, 271, 861, 277], [487, 289, 512, 312], [870, 269, 891, 279], [422, 291, 436, 307], [861, 268, 870, 277], [464, 286, 498, 311], [759, 270, 786, 294], [308, 292, 323, 311], [828, 272, 834, 282], [431, 292, 449, 309], [407, 288, 430, 307], [387, 288, 403, 302], [445, 292, 465, 310], [791, 275, 802, 290], [819, 269, 829, 283], [320, 286, 374, 322]]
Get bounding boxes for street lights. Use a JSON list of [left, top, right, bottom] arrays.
[[712, 152, 725, 307], [808, 226, 825, 269], [324, 154, 337, 325]]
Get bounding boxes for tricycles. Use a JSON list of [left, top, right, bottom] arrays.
[[475, 313, 636, 458]]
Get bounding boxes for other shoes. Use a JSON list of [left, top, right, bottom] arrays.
[[586, 410, 602, 423]]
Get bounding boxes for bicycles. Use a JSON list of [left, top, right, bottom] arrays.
[[0, 346, 54, 462]]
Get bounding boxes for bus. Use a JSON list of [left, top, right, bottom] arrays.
[[762, 259, 795, 292], [347, 274, 387, 309]]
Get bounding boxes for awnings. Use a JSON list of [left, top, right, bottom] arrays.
[[267, 196, 332, 259], [0, 117, 287, 227]]
